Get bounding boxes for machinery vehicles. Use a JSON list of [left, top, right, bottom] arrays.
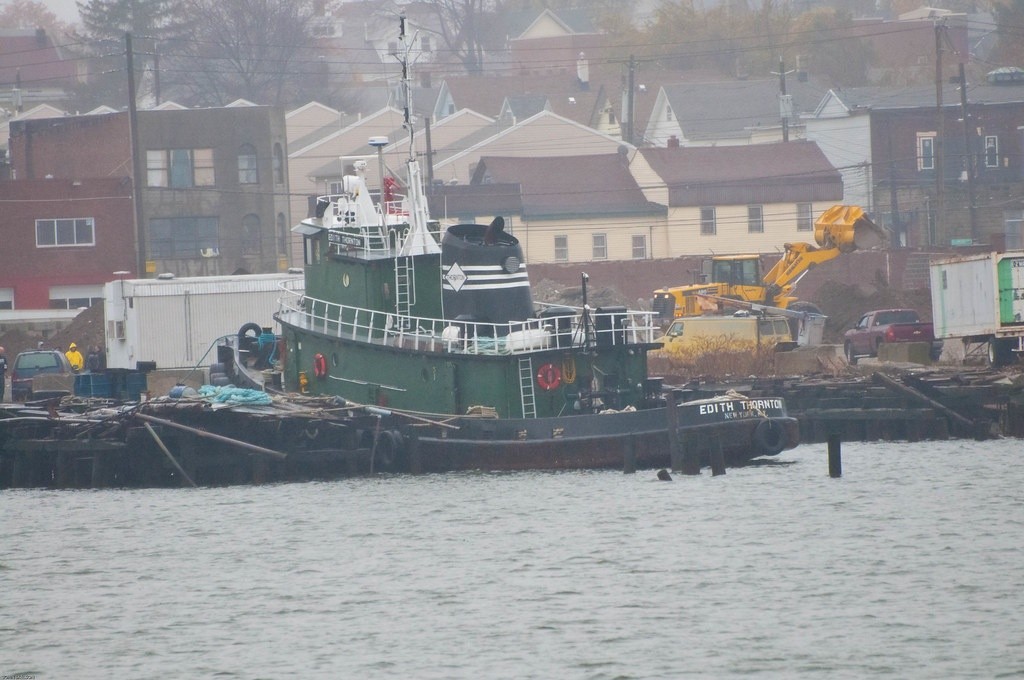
[[651, 203, 887, 330]]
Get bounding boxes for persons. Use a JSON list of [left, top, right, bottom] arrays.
[[0, 347, 7, 403], [65, 342, 83, 370], [85, 345, 105, 369]]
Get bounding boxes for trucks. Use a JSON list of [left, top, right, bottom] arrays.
[[930, 251, 1024, 366]]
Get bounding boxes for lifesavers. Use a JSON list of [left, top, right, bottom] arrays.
[[313, 353, 326, 378], [537, 362, 562, 391], [239, 322, 262, 337], [754, 418, 786, 456], [378, 430, 404, 473]]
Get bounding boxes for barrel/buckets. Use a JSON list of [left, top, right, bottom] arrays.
[[74, 362, 156, 400], [169, 386, 198, 398]]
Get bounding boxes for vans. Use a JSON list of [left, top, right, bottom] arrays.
[[11, 351, 78, 397], [647, 316, 792, 366]]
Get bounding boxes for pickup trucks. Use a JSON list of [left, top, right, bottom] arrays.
[[844, 308, 933, 366]]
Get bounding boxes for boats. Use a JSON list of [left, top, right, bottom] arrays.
[[209, 11, 800, 468]]
